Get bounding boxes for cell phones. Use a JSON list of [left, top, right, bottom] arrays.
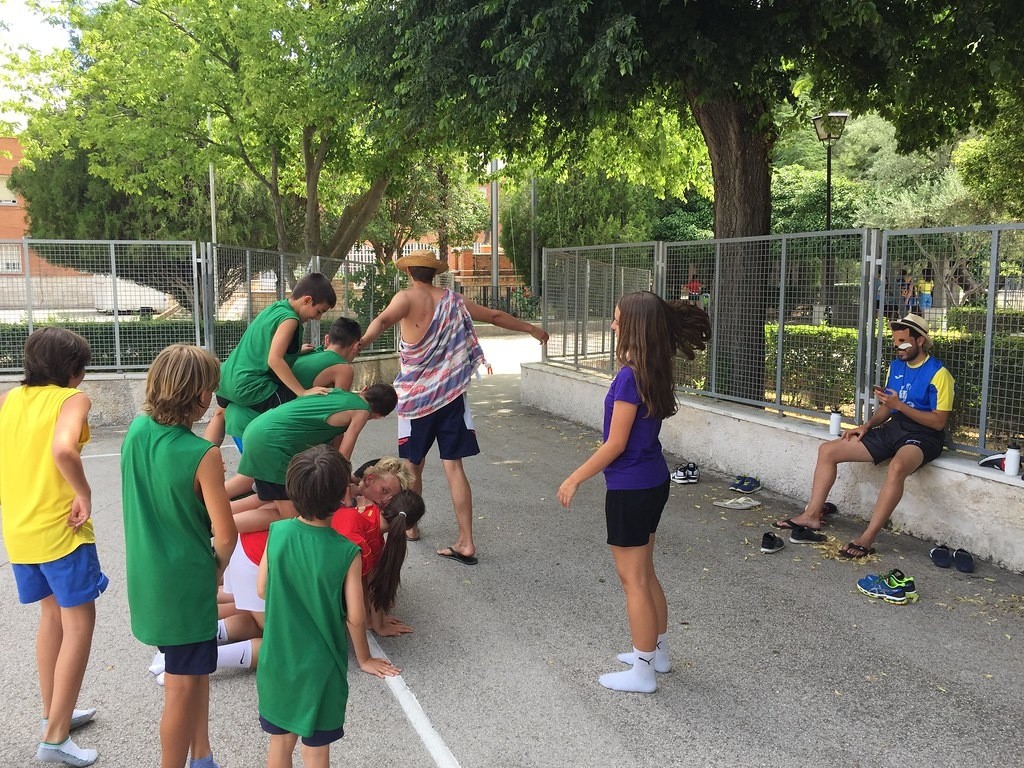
[[872, 385, 883, 394]]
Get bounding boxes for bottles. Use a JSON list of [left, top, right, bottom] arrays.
[[829, 406, 841, 435], [1005, 442, 1020, 478]]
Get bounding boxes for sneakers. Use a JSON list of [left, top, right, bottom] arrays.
[[760, 532, 785, 554], [728, 473, 748, 491], [978, 453, 1024, 472], [790, 527, 828, 543], [736, 476, 762, 494], [805, 501, 838, 516], [670, 463, 687, 484], [856, 576, 907, 605], [819, 517, 829, 525], [865, 569, 918, 598], [686, 462, 700, 484]]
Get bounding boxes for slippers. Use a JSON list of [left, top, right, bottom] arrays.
[[404, 529, 420, 542], [952, 548, 974, 573], [927, 545, 951, 568], [772, 518, 822, 531], [723, 497, 762, 506], [712, 498, 752, 510], [838, 543, 875, 558], [436, 547, 479, 565]]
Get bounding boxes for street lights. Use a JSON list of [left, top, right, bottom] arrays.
[[810, 96, 849, 325]]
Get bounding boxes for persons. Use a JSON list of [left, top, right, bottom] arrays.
[[149, 457, 425, 686], [918, 269, 934, 310], [225, 316, 361, 499], [0, 326, 109, 768], [771, 313, 956, 558], [204, 273, 336, 449], [897, 270, 916, 314], [119, 344, 239, 768], [557, 291, 712, 692], [230, 384, 399, 535], [685, 274, 703, 308], [256, 444, 367, 768], [360, 249, 549, 565]]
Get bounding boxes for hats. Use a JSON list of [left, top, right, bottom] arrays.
[[394, 249, 449, 275], [889, 313, 933, 351]]
